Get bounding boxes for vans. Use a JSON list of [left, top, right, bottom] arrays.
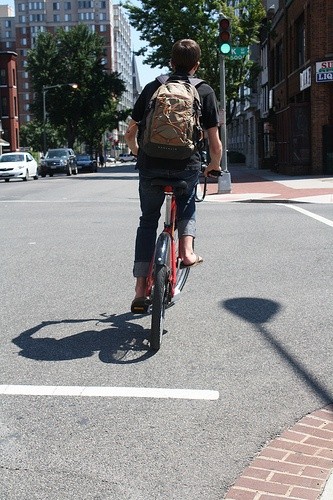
[[118, 154, 134, 163]]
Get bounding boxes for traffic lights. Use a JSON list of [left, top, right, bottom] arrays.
[[219, 18, 231, 56]]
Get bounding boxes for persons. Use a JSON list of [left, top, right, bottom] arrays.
[[124, 39, 222, 311]]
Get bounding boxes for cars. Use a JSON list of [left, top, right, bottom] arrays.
[[76, 155, 97, 173], [0, 152, 38, 182]]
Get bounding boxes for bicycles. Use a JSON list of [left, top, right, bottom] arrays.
[[129, 151, 223, 350]]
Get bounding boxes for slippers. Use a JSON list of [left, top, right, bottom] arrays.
[[131, 297, 148, 314], [181, 254, 203, 269]]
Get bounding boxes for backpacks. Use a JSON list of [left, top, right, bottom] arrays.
[[138, 75, 206, 161]]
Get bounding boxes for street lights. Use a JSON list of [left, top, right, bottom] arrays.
[[42, 84, 78, 156]]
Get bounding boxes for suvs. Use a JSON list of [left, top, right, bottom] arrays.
[[40, 148, 77, 177]]
